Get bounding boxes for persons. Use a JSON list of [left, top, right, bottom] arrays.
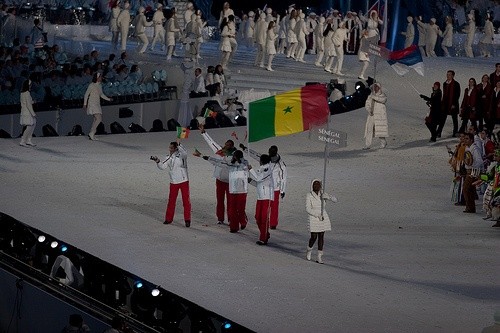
[[84, 72, 114, 141], [0, 0, 500, 246], [20, 80, 37, 147], [305, 178, 337, 264], [364, 82, 389, 149]]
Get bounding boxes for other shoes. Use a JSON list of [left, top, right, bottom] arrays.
[[257, 241, 267, 245], [163, 221, 172, 224], [456, 202, 500, 228], [229, 230, 236, 233], [379, 142, 387, 149], [149, 45, 365, 80], [241, 225, 245, 230], [218, 221, 222, 224], [185, 221, 190, 227]]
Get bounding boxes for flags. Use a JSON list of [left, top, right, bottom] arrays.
[[204, 108, 217, 117], [248, 84, 330, 143], [387, 44, 425, 77], [176, 126, 189, 140]]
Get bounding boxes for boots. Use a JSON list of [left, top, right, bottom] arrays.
[[306, 245, 313, 261], [88, 128, 97, 140], [19, 137, 33, 146], [316, 250, 324, 264]]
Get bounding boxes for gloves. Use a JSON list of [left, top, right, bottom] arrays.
[[318, 214, 323, 221], [322, 192, 330, 199], [281, 192, 286, 198]]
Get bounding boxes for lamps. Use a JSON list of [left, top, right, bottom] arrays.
[[96, 122, 105, 135], [110, 122, 127, 134], [0, 129, 12, 139], [68, 125, 85, 136], [43, 124, 59, 137], [18, 126, 35, 137], [328, 81, 369, 115], [129, 123, 146, 134], [233, 114, 247, 126], [149, 120, 163, 132], [167, 118, 178, 132], [223, 319, 234, 330]]
